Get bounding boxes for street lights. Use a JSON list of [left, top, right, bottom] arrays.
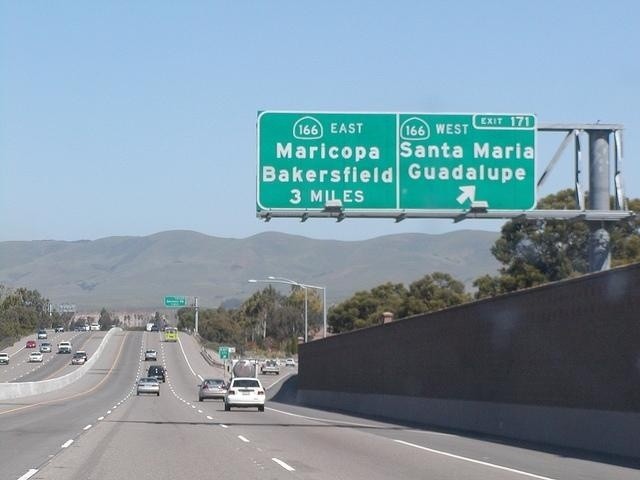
[[248, 276, 326, 343]]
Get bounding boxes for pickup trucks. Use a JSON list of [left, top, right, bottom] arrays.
[[262, 360, 280, 374]]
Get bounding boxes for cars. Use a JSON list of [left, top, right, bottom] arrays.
[[137, 350, 167, 396], [164, 327, 178, 342], [285, 358, 295, 366], [151, 325, 159, 332], [0, 325, 87, 365], [197, 377, 265, 412]]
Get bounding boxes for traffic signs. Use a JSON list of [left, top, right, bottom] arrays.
[[255, 111, 535, 213]]
